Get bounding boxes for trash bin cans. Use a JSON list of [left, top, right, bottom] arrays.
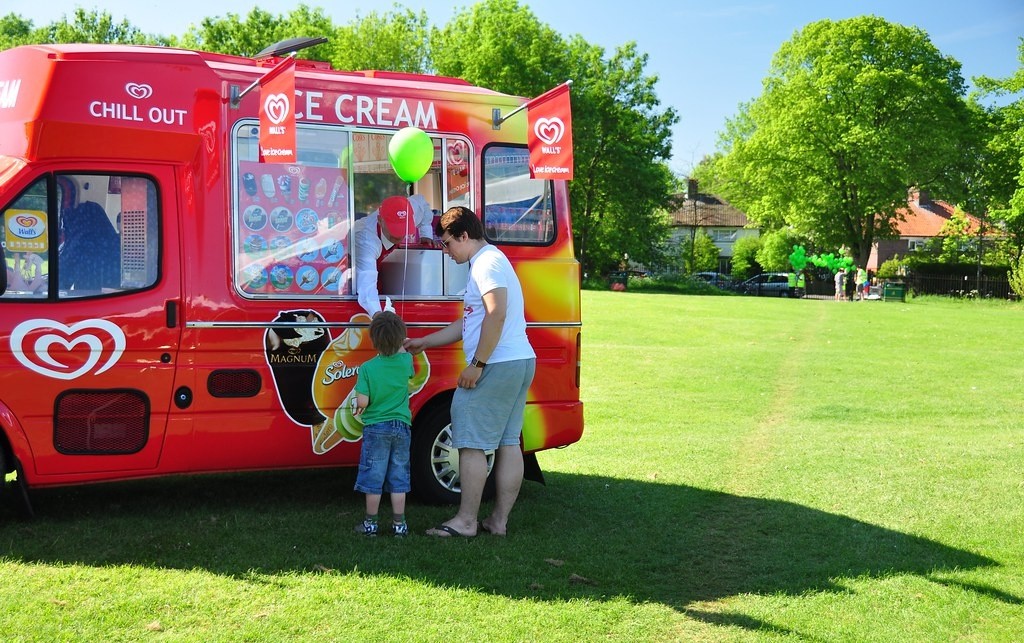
[[883, 281, 907, 304]]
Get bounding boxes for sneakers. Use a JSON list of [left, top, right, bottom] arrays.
[[353, 520, 379, 536], [392, 524, 408, 538]]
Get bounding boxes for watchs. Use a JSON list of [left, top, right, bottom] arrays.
[[471, 356, 486, 369]]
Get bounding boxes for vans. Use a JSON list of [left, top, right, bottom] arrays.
[[734, 272, 789, 298]]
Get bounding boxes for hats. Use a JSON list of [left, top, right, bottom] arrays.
[[379, 195, 416, 236]]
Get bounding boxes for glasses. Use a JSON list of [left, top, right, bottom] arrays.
[[440, 232, 458, 248]]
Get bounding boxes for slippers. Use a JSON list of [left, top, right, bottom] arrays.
[[476, 518, 492, 532], [424, 525, 477, 539]]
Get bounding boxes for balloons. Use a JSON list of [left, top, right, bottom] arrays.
[[789, 244, 856, 275], [388, 127, 434, 183]]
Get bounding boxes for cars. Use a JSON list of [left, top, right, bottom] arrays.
[[694, 272, 732, 290], [609, 271, 651, 284]]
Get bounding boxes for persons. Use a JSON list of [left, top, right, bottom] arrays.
[[834, 268, 847, 302], [353, 191, 433, 319], [641, 266, 652, 277], [619, 253, 631, 277], [787, 268, 798, 298], [57, 198, 122, 289], [403, 207, 538, 539], [855, 264, 876, 301], [797, 269, 805, 298], [353, 312, 415, 538]]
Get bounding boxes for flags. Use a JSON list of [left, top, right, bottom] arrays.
[[527, 83, 574, 181], [259, 55, 296, 163]]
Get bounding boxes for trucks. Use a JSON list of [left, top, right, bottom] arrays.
[[0, 36, 584, 506]]
